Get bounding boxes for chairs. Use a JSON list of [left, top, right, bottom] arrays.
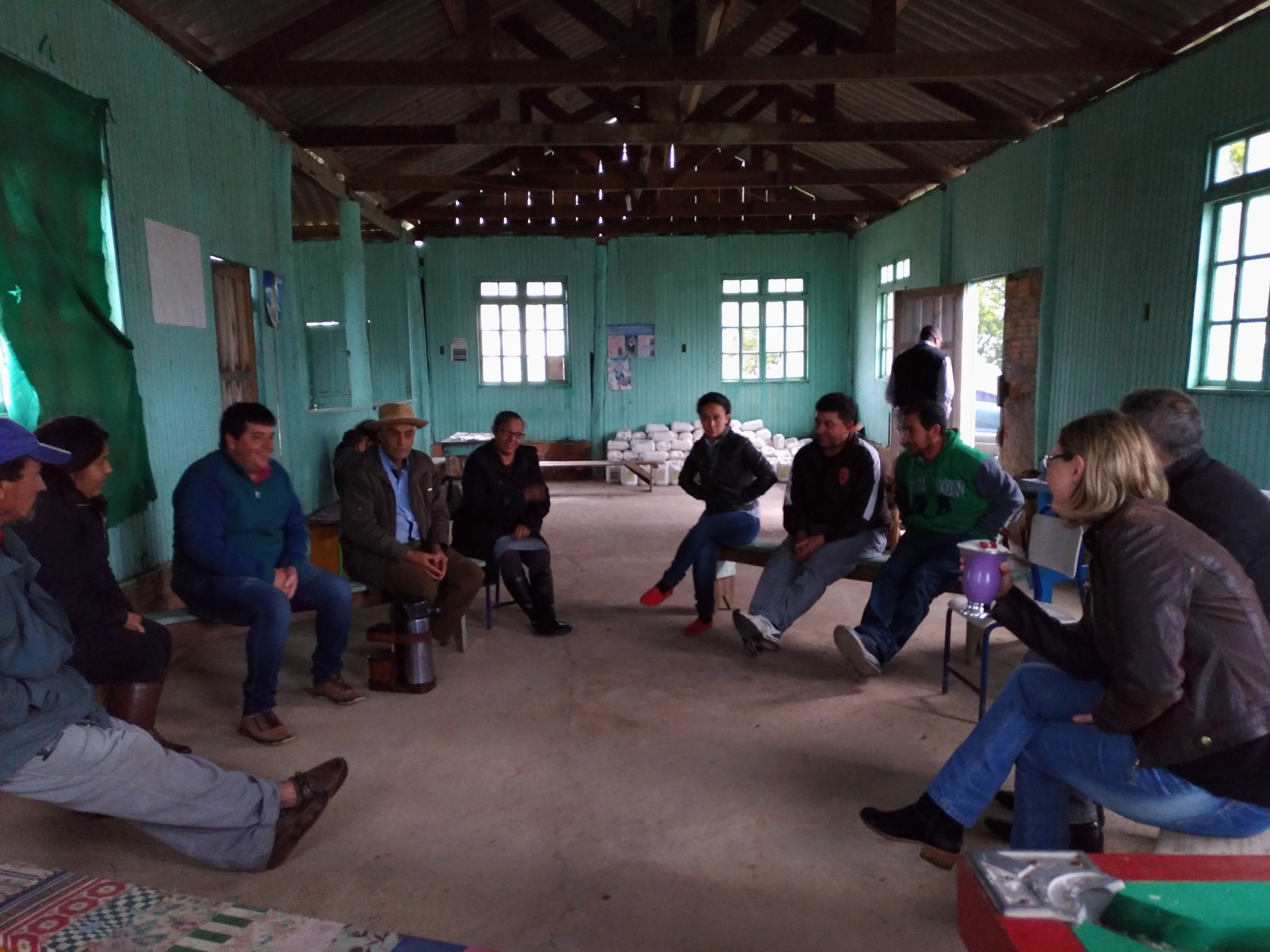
[[941, 504, 1089, 723]]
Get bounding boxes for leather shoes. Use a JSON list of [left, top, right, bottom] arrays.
[[993, 789, 1105, 829], [983, 817, 1104, 854]]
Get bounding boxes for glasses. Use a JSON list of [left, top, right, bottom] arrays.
[[501, 426, 526, 440], [1044, 450, 1066, 468]]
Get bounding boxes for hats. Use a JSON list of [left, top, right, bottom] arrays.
[[0, 417, 70, 469], [33, 415, 109, 473], [362, 403, 429, 429]]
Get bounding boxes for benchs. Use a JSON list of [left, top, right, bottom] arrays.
[[538, 458, 667, 492], [709, 539, 993, 667], [143, 554, 486, 652]]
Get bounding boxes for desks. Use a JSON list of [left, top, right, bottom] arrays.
[[441, 432, 495, 481]]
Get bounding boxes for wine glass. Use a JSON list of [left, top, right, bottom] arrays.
[[956, 538, 1013, 618]]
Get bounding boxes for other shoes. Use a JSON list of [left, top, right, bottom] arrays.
[[683, 617, 713, 636], [238, 707, 295, 746], [640, 581, 674, 606], [261, 790, 329, 870], [288, 756, 348, 807], [312, 673, 365, 705]]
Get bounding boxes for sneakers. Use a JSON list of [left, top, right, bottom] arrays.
[[732, 607, 781, 657], [834, 625, 882, 678]]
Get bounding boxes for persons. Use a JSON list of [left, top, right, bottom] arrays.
[[732, 393, 892, 653], [884, 324, 955, 420], [856, 408, 1269, 869], [834, 400, 1025, 682], [333, 417, 375, 496], [985, 387, 1270, 852], [0, 418, 348, 873], [339, 402, 485, 646], [452, 410, 572, 636], [19, 415, 191, 754], [169, 401, 368, 744], [639, 393, 779, 636]]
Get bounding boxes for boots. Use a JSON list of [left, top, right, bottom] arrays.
[[529, 567, 573, 634], [106, 677, 194, 757], [504, 570, 540, 632], [860, 791, 965, 871]]
